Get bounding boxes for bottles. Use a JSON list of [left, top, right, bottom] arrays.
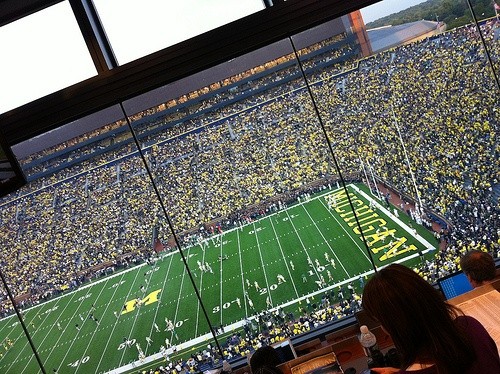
[[360, 326, 383, 369]]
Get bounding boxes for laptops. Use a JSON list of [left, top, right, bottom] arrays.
[[438, 270, 473, 301]]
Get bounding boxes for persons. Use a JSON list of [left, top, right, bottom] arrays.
[[4, 11, 499, 374]]
